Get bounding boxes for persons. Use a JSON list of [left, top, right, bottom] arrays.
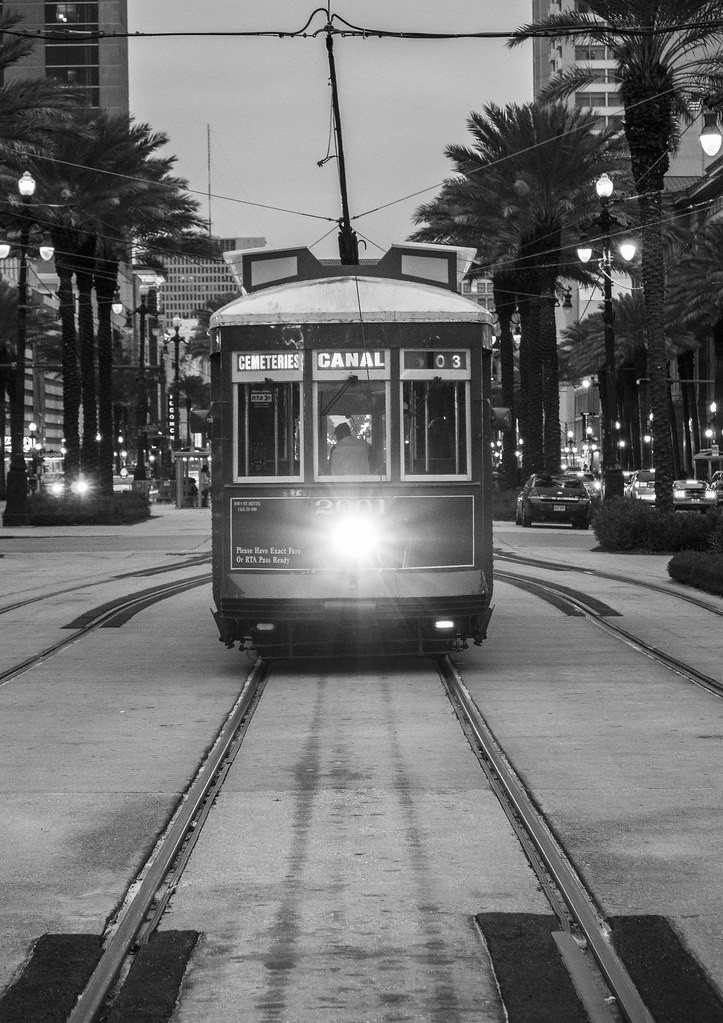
[[28, 473, 39, 494], [189, 478, 199, 507], [326, 422, 379, 475], [201, 465, 211, 507]]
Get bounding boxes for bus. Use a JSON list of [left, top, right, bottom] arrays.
[[211, 35, 511, 668]]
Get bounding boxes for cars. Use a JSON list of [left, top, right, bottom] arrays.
[[44, 473, 200, 504], [563, 465, 723, 512]]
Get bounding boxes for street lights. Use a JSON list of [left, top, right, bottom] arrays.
[[163, 314, 194, 500], [111, 279, 162, 507], [565, 431, 577, 465], [0, 168, 54, 526], [516, 472, 591, 530], [577, 173, 637, 499], [29, 423, 37, 449]]
